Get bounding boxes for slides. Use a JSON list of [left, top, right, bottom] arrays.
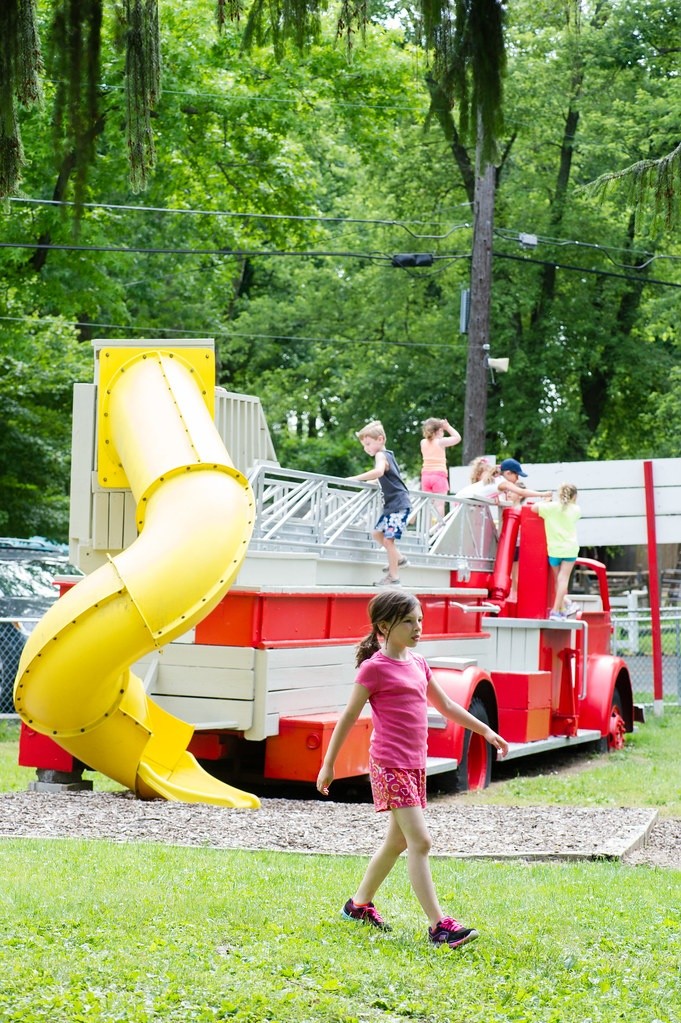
[[13, 346, 264, 811]]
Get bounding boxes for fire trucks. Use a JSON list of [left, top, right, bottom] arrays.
[[10, 336, 643, 810]]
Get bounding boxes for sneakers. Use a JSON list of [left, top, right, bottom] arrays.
[[550, 609, 566, 620], [428, 915, 479, 949], [562, 602, 582, 615], [382, 555, 410, 571], [341, 898, 394, 935], [373, 576, 401, 587]]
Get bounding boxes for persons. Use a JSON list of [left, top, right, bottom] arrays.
[[532, 483, 582, 618], [451, 458, 529, 514], [317, 590, 510, 948], [469, 458, 492, 483], [421, 417, 462, 534], [345, 420, 414, 586], [507, 481, 527, 506]]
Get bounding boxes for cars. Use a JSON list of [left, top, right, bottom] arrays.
[[0, 549, 84, 714]]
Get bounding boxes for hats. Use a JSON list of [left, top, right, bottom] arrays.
[[499, 458, 528, 478]]
